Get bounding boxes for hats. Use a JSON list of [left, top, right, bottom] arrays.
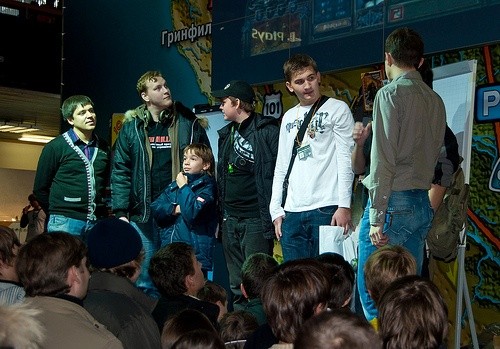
[[210, 80, 258, 104], [87, 214, 143, 269]]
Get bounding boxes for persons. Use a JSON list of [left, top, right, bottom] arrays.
[[147, 242, 261, 349], [110, 70, 215, 297], [357, 26, 446, 318], [364, 244, 417, 334], [351, 66, 464, 320], [32, 96, 110, 241], [149, 143, 217, 280], [269, 54, 356, 262], [20, 194, 46, 242], [379, 274, 448, 349], [260, 260, 330, 349], [83, 218, 161, 349], [211, 80, 280, 297], [294, 310, 379, 349], [0, 231, 123, 349], [234, 253, 280, 349], [0, 225, 25, 305], [317, 252, 355, 311]]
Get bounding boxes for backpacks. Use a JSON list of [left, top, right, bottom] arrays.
[[426, 165, 470, 261]]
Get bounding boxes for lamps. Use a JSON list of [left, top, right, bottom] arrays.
[[0, 117, 41, 133]]
